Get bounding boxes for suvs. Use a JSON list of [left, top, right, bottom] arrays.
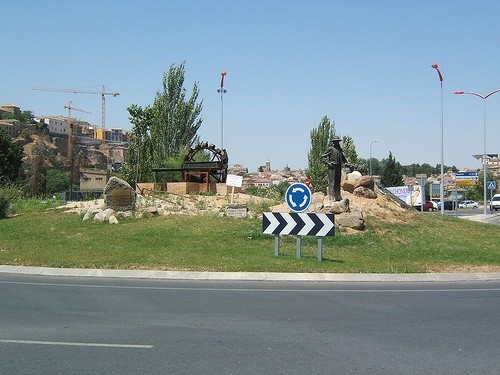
[[490, 194, 500, 211]]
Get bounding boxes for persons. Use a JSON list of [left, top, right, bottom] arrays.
[[322, 136, 348, 201]]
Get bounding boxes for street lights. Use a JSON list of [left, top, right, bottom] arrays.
[[370, 139, 378, 176], [217, 72, 228, 148], [431, 64, 444, 216], [453, 89, 500, 215]]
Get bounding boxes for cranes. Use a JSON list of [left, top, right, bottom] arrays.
[[32, 85, 120, 140], [64, 100, 93, 118]]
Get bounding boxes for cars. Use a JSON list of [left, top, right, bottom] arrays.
[[416, 198, 459, 212], [458, 200, 479, 210]]
